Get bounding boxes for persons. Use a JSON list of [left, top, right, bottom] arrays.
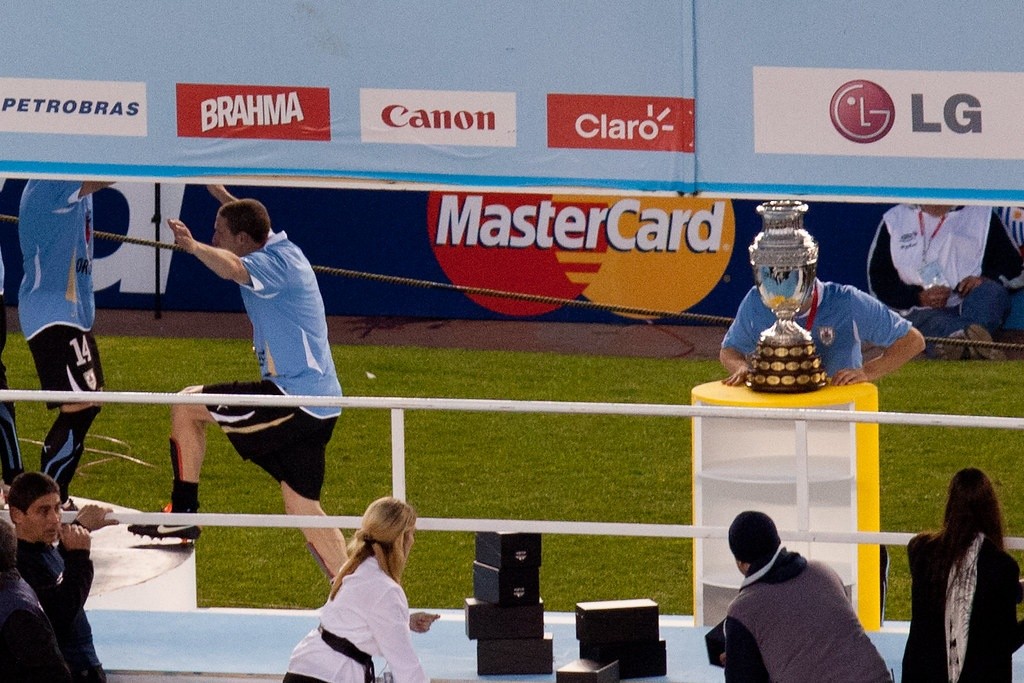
[[718, 270, 926, 389], [125, 182, 348, 577], [723, 509, 897, 682], [0, 517, 71, 682], [14, 179, 118, 511], [11, 471, 119, 683], [900, 467, 1022, 683], [279, 498, 440, 682], [867, 202, 1023, 362]]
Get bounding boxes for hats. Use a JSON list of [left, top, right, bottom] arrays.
[[728, 510, 781, 563]]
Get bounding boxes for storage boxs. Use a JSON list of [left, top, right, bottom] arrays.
[[458, 530, 667, 683]]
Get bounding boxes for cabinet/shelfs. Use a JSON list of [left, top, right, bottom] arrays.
[[689, 381, 883, 633]]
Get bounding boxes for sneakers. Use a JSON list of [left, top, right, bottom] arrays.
[[936, 330, 966, 361], [55, 501, 81, 524], [965, 324, 1006, 362], [126, 521, 200, 550]]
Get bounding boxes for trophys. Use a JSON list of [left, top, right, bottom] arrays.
[[747, 199, 830, 396]]
